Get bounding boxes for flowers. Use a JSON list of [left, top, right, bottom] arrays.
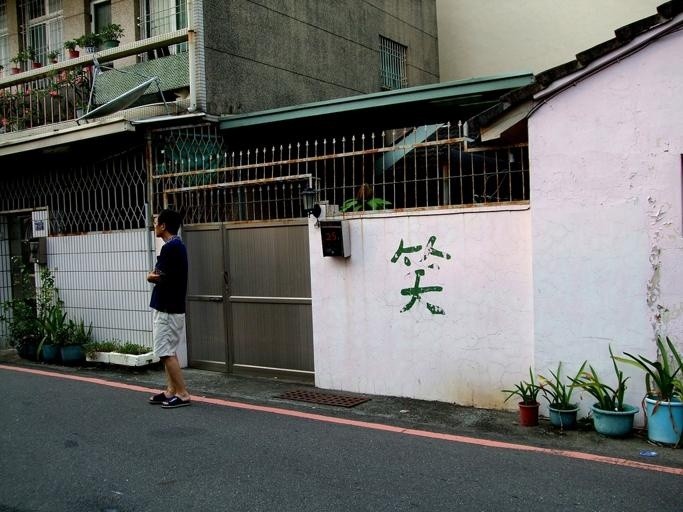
[[0, 69, 86, 124]]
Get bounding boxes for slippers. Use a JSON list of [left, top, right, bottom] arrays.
[[161, 395, 192, 408], [149, 392, 176, 404]]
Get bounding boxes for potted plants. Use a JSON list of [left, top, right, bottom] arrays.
[[3, 261, 159, 375], [501, 338, 683, 449], [8, 22, 125, 76]]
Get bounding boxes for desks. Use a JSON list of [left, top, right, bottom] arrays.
[[93, 52, 189, 107]]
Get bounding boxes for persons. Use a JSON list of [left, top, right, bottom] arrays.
[[145, 209, 193, 409]]
